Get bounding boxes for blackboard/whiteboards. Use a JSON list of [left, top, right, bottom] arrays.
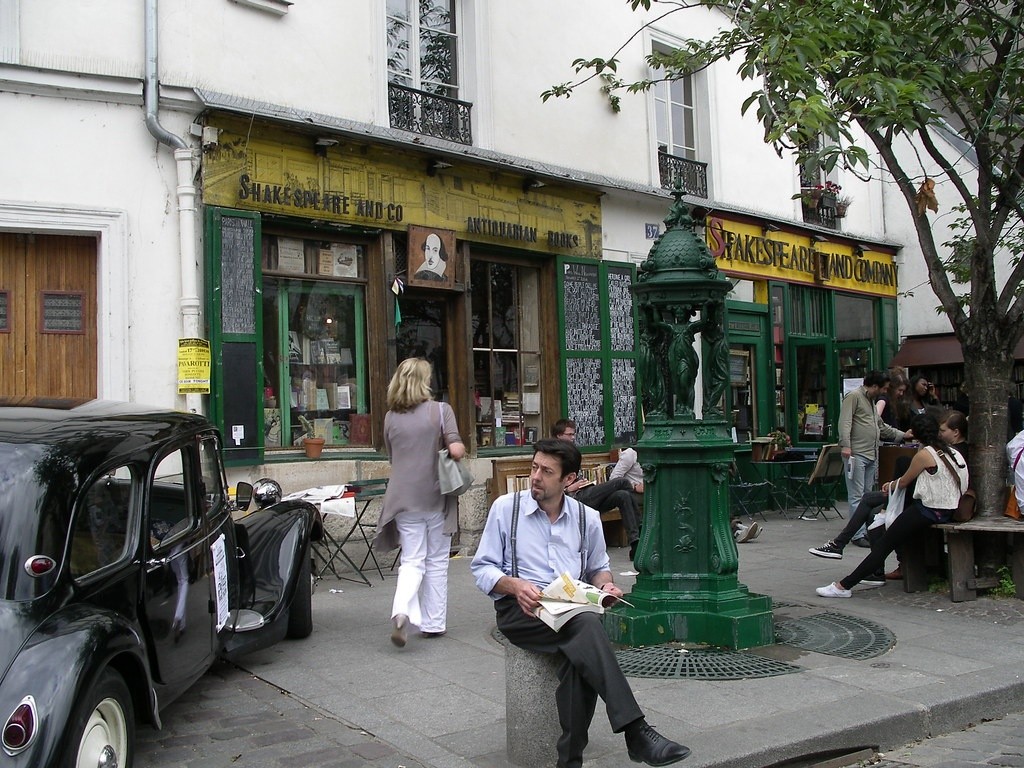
[[608, 265, 635, 352], [223, 215, 256, 333], [612, 358, 639, 444], [564, 262, 602, 350], [221, 341, 260, 460], [566, 358, 604, 445]]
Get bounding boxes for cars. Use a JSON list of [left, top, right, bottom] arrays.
[[0, 406, 325, 768]]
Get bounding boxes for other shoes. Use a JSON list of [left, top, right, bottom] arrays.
[[629, 550, 636, 560]]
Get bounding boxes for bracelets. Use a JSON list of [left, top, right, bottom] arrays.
[[600, 584, 605, 590]]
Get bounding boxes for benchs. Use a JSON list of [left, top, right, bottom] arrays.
[[77, 481, 184, 534], [899, 514, 1024, 604], [485, 452, 628, 548]]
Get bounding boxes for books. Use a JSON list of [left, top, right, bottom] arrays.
[[579, 479, 597, 489], [501, 391, 525, 424], [531, 570, 636, 634]]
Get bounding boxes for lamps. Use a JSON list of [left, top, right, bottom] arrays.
[[767, 223, 782, 232], [855, 245, 871, 252], [316, 137, 340, 148], [813, 235, 830, 243], [432, 160, 455, 170], [528, 179, 548, 190]]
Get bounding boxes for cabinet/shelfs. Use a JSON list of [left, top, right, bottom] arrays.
[[291, 362, 357, 421]]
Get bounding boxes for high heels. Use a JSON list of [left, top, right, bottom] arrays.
[[422, 629, 448, 639], [390, 615, 409, 648]]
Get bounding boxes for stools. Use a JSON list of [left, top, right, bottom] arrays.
[[503, 637, 561, 768]]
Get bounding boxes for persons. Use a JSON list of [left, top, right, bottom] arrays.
[[371, 357, 465, 648], [470, 439, 692, 768], [549, 419, 763, 561], [167, 541, 190, 640], [807, 364, 1024, 598]]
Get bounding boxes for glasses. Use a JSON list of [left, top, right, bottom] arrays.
[[565, 433, 575, 436]]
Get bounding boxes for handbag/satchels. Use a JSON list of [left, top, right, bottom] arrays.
[[953, 489, 978, 523], [1003, 485, 1022, 521], [438, 448, 475, 497]]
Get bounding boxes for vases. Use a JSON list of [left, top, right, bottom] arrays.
[[817, 194, 835, 209], [771, 451, 788, 462]]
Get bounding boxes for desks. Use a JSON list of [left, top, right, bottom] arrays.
[[310, 495, 374, 589], [748, 459, 818, 522]]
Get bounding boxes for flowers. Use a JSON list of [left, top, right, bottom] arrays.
[[767, 430, 793, 450], [814, 181, 842, 197]]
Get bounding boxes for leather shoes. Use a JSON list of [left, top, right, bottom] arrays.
[[625, 717, 691, 767]]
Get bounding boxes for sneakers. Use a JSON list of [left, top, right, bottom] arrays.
[[736, 522, 763, 543], [850, 534, 871, 548], [816, 582, 852, 597], [809, 540, 843, 559], [853, 580, 886, 590], [886, 566, 904, 579]]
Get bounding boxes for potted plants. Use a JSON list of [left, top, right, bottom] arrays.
[[297, 415, 326, 458], [834, 195, 853, 216], [792, 190, 820, 209]]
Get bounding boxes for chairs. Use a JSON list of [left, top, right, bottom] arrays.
[[729, 463, 768, 521], [797, 443, 845, 522], [782, 447, 819, 510], [348, 478, 402, 581]]
